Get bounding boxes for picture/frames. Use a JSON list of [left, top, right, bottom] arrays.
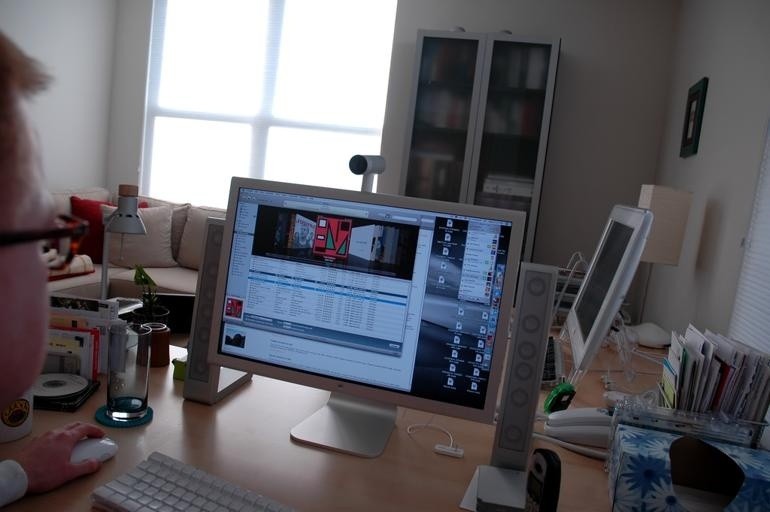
[[680, 76, 709, 157]]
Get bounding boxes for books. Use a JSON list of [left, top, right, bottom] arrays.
[[656, 326, 770, 422]]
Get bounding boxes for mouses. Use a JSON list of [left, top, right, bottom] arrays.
[[68, 437, 119, 463]]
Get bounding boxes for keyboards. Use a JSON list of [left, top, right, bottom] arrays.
[[88, 448, 300, 512], [540, 335, 564, 389]]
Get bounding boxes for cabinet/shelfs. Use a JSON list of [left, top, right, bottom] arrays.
[[398, 27, 562, 308]]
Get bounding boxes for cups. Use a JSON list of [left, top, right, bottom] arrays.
[[106, 323, 152, 422], [0, 391, 35, 444]]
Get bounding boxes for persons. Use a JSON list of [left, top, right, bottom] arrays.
[[0, 31, 104, 500], [291, 224, 315, 250]]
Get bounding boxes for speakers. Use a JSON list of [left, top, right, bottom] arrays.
[[489, 260, 560, 473], [181, 215, 253, 407]]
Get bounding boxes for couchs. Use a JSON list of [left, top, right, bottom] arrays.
[[44, 187, 227, 299]]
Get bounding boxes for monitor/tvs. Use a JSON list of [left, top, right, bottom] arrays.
[[205, 174, 528, 460], [566, 202, 656, 372]]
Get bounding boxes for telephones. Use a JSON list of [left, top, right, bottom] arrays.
[[545, 408, 614, 449]]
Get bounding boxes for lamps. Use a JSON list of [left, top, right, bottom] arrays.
[[629, 184, 692, 348], [98, 184, 148, 301]]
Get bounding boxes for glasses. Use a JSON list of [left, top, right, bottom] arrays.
[[0, 210, 89, 273]]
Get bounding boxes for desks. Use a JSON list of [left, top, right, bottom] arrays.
[[0, 328, 728, 512]]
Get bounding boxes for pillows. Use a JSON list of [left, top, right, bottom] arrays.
[[46, 190, 228, 270]]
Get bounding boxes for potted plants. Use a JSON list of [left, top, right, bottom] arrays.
[[130, 264, 170, 332]]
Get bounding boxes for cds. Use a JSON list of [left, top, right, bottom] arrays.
[[33, 372, 89, 398]]
